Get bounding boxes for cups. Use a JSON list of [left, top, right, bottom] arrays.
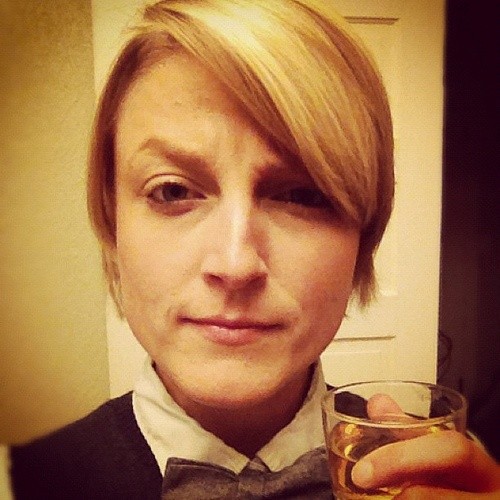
[[321, 380, 466, 499]]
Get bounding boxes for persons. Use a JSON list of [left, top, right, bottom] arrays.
[[0, 0, 500, 500]]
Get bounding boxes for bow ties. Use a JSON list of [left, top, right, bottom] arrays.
[[160, 444, 328, 498]]
